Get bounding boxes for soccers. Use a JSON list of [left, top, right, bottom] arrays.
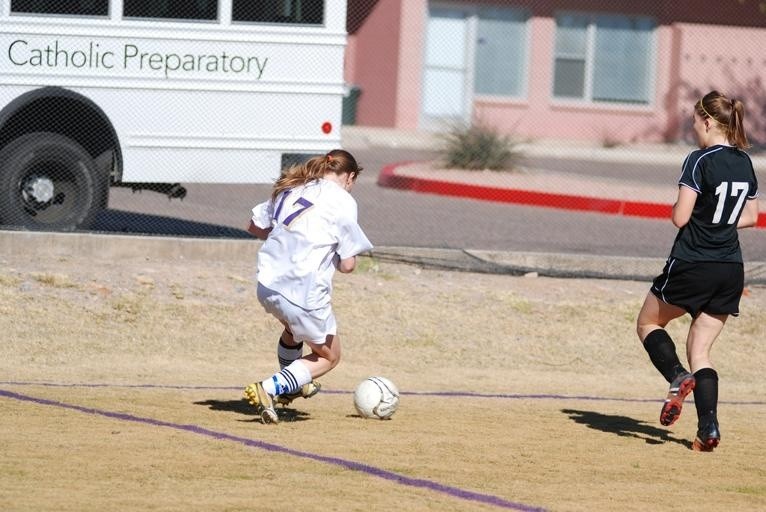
[[354, 374, 402, 421]]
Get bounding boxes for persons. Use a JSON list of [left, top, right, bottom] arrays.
[[241, 148, 374, 425], [637, 90, 760, 451]]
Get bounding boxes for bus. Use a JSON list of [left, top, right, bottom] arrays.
[[0, 2, 347, 232]]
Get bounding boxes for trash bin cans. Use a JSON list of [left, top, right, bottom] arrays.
[[342, 87, 361, 125]]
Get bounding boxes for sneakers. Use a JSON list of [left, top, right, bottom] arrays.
[[692, 417, 720, 452], [660, 370, 696, 427], [243, 382, 279, 425], [273, 379, 321, 407]]
